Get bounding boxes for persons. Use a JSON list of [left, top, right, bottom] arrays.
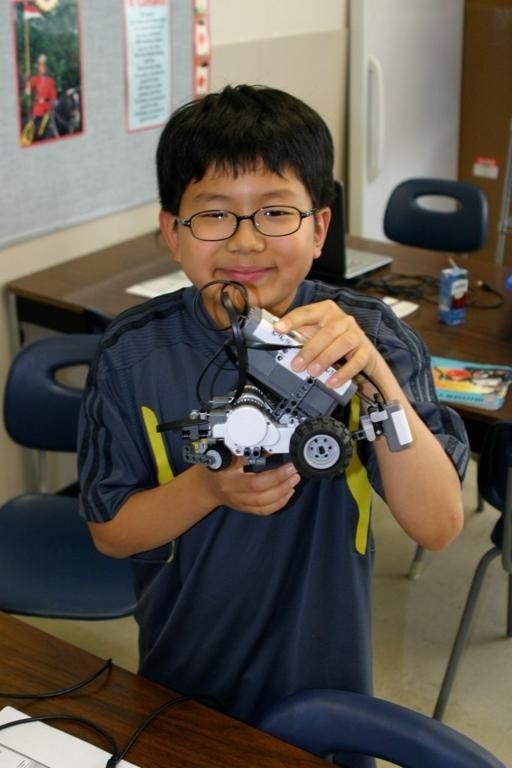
[[78, 83, 469, 768], [23, 52, 60, 141]]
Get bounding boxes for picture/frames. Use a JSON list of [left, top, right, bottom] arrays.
[[9, 0, 86, 148], [124, 4, 173, 133]]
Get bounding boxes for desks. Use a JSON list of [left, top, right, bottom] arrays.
[[0, 613, 334, 768], [8, 233, 512, 425]]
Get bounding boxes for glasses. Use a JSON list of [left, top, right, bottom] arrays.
[[171, 205, 318, 242]]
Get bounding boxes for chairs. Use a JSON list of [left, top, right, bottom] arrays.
[[1, 334, 175, 621], [258, 690, 506, 765], [382, 176, 488, 254], [427, 421, 512, 720]]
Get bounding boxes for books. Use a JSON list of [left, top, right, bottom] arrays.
[[427, 355, 511, 411]]
